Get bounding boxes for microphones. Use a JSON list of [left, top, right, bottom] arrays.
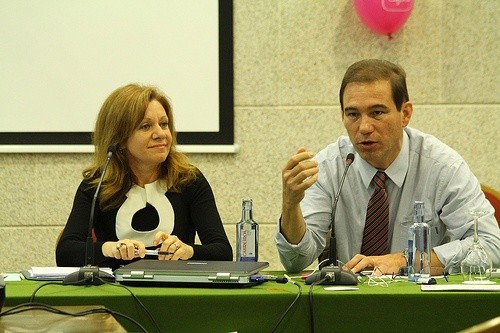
[[63, 145, 117, 286], [305, 153, 358, 285]]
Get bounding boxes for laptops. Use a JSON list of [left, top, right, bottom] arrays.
[[113, 259, 270, 287]]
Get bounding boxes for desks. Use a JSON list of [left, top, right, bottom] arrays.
[[0, 271, 500, 333]]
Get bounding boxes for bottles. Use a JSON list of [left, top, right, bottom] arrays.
[[236, 200, 259, 262], [407, 200, 432, 283]]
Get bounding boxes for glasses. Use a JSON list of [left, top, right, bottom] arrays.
[[392, 267, 448, 282]]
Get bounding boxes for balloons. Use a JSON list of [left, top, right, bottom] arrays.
[[354, 0, 415, 40]]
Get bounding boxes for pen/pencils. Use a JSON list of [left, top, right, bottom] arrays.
[[135, 249, 174, 255]]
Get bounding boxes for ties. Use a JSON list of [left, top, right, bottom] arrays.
[[360, 172, 390, 256]]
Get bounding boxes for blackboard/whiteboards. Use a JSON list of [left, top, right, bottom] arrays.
[[0, 0, 237, 154]]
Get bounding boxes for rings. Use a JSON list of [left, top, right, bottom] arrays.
[[372, 258, 374, 262], [173, 243, 178, 250], [117, 242, 121, 248]]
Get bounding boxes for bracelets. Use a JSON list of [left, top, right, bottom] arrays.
[[402, 248, 408, 266]]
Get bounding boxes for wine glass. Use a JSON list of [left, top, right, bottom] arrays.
[[459, 208, 493, 281]]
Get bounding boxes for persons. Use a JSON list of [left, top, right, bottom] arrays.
[[56, 83, 233, 269], [274, 58, 500, 275]]
